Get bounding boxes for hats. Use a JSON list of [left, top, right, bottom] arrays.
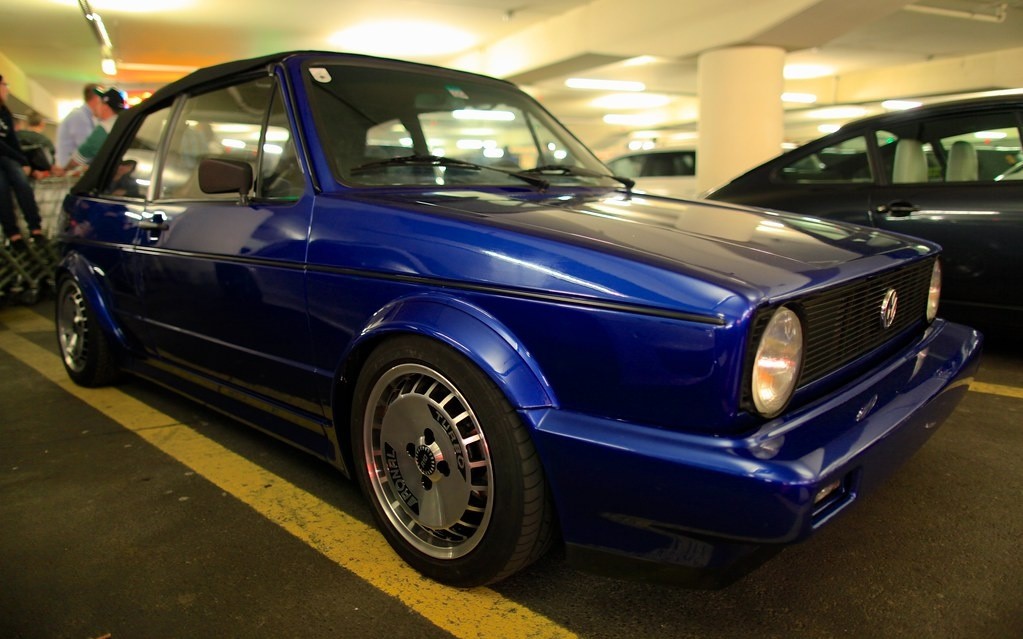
[[92, 87, 125, 114]]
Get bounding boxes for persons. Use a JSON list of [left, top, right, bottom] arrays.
[[105, 159, 139, 198], [0, 74, 50, 253], [14, 110, 55, 179], [50, 88, 124, 173], [54, 83, 106, 175]]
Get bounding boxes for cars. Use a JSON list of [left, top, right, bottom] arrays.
[[0, 108, 228, 254], [54, 46, 982, 590], [700, 87, 1023, 332], [607, 143, 823, 197]]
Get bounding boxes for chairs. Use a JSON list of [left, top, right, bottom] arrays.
[[945, 142, 978, 181], [286, 123, 366, 157], [894, 139, 927, 184]]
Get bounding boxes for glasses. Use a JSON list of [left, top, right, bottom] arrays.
[[0, 82, 10, 88]]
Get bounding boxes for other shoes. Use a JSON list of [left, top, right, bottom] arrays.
[[10, 238, 28, 254], [30, 231, 47, 245]]
